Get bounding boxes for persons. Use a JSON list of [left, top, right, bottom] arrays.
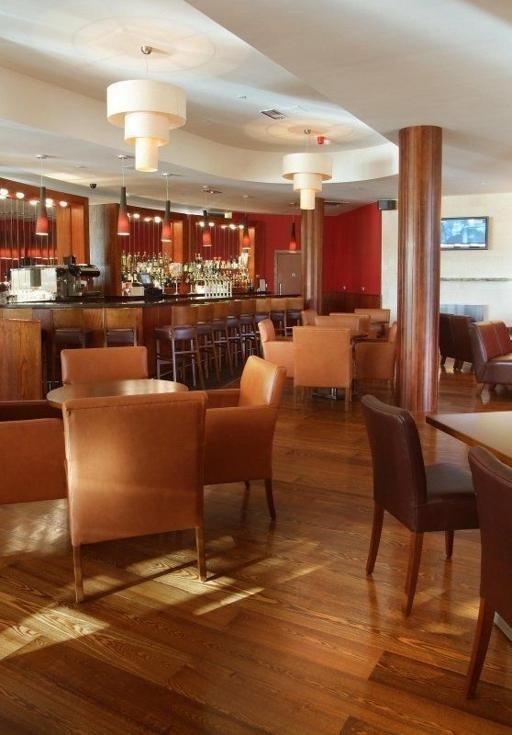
[[446, 222, 463, 244]]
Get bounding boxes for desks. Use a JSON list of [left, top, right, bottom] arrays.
[[46, 378, 189, 408], [426, 409, 512, 461]]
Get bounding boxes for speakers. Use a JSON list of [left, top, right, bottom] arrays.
[[379, 200, 396, 210]]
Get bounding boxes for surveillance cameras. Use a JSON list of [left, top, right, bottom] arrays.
[[90, 184, 96, 189]]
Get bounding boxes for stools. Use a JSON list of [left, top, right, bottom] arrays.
[[154, 296, 303, 388], [4, 308, 143, 382]]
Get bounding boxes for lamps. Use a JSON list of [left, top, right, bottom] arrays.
[[241, 194, 253, 250], [34, 153, 50, 236], [105, 44, 188, 177], [201, 188, 213, 248], [286, 202, 297, 254], [158, 171, 175, 243], [281, 127, 333, 211], [115, 153, 134, 236]]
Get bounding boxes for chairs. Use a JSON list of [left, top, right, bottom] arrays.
[[360, 394, 480, 617], [2, 400, 68, 538], [258, 319, 292, 386], [60, 346, 149, 380], [62, 391, 207, 603], [462, 444, 509, 702], [437, 313, 475, 370], [195, 355, 287, 523], [468, 319, 510, 397], [300, 308, 390, 336], [292, 326, 353, 409], [354, 320, 398, 393]]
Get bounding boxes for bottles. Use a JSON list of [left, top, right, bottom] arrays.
[[232, 273, 247, 290], [121, 249, 171, 283], [184, 253, 246, 273]]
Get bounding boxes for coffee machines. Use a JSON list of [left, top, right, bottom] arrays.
[[10, 256, 102, 299]]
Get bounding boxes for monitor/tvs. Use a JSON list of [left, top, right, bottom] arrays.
[[441, 216, 488, 250], [136, 272, 153, 285]]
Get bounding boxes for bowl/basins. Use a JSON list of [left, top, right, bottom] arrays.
[[165, 288, 175, 294]]
